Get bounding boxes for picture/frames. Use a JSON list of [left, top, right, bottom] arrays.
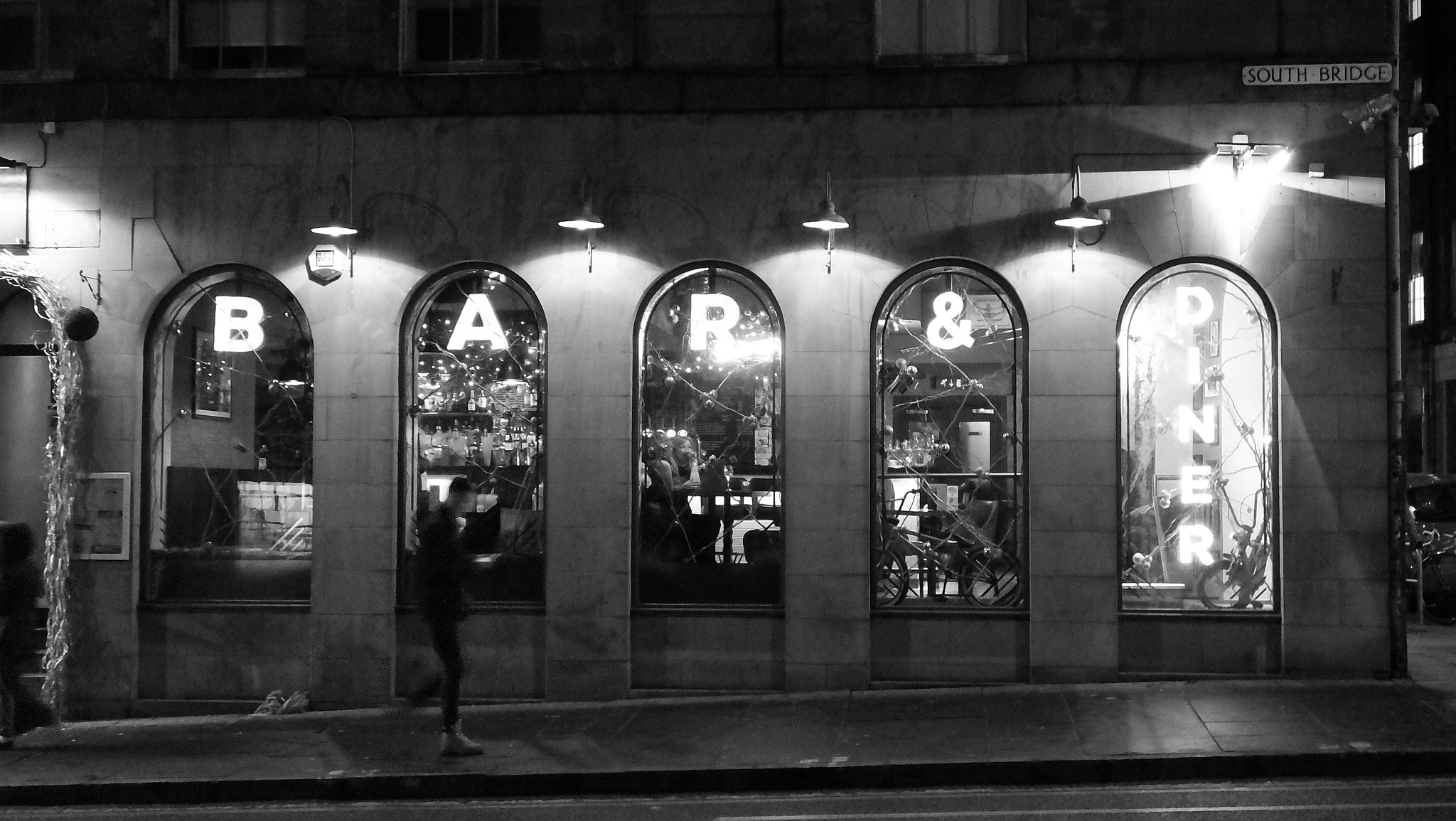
[[67, 469, 132, 563], [190, 325, 234, 425]]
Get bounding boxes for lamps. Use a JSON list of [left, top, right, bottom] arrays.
[[309, 172, 360, 247], [556, 166, 605, 250], [1053, 161, 1104, 253], [800, 161, 851, 250]]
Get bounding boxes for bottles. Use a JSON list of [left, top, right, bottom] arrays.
[[524, 384, 531, 408], [461, 417, 487, 465], [419, 420, 461, 435], [459, 389, 467, 412], [514, 427, 543, 466], [531, 384, 537, 407], [478, 390, 488, 413], [452, 390, 458, 412], [467, 390, 477, 413], [435, 392, 452, 413], [492, 426, 513, 466]]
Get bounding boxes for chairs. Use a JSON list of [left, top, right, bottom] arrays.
[[930, 496, 1003, 601], [698, 475, 783, 565]]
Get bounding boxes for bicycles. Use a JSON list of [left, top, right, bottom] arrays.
[[872, 489, 1025, 610], [1197, 478, 1270, 609]]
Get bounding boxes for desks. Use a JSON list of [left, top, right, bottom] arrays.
[[666, 486, 754, 571], [883, 504, 967, 598]]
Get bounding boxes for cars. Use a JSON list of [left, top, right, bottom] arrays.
[[1402, 471, 1456, 626]]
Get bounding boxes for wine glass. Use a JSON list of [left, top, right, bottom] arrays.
[[724, 466, 733, 491]]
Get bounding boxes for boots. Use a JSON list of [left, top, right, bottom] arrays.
[[441, 719, 482, 753]]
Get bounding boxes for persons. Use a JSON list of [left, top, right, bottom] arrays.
[[419, 479, 483, 757], [640, 432, 721, 565]]
